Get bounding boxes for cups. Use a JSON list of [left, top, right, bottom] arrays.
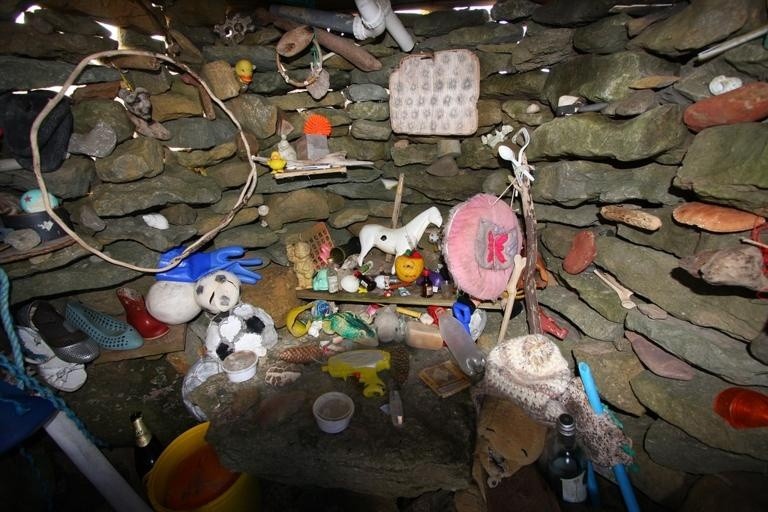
[[440, 280, 454, 300], [306, 133, 330, 162], [221, 350, 259, 383], [312, 391, 355, 435]]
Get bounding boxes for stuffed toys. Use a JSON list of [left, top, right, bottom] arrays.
[[469, 398, 560, 512]]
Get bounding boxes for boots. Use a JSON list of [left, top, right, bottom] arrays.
[[117, 287, 170, 340]]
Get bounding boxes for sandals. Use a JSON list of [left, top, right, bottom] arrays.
[[15, 325, 89, 393], [65, 299, 143, 351]]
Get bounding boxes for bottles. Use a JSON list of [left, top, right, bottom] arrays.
[[326, 258, 340, 295], [541, 414, 589, 511], [132, 409, 163, 482], [355, 270, 376, 293], [422, 270, 433, 298], [434, 307, 487, 379]]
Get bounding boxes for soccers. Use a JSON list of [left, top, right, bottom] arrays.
[[205, 304, 280, 363], [182, 357, 222, 422]]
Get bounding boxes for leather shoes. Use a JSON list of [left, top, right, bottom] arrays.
[[17, 300, 100, 363]]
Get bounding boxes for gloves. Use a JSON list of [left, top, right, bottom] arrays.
[[155, 247, 262, 284]]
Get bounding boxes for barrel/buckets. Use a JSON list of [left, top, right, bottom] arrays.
[[146, 422, 247, 511]]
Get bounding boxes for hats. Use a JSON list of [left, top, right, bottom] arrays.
[[471, 335, 572, 431]]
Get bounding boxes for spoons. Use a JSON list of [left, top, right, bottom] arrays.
[[497, 143, 536, 183]]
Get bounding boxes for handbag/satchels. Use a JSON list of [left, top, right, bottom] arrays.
[[473, 393, 548, 502]]
